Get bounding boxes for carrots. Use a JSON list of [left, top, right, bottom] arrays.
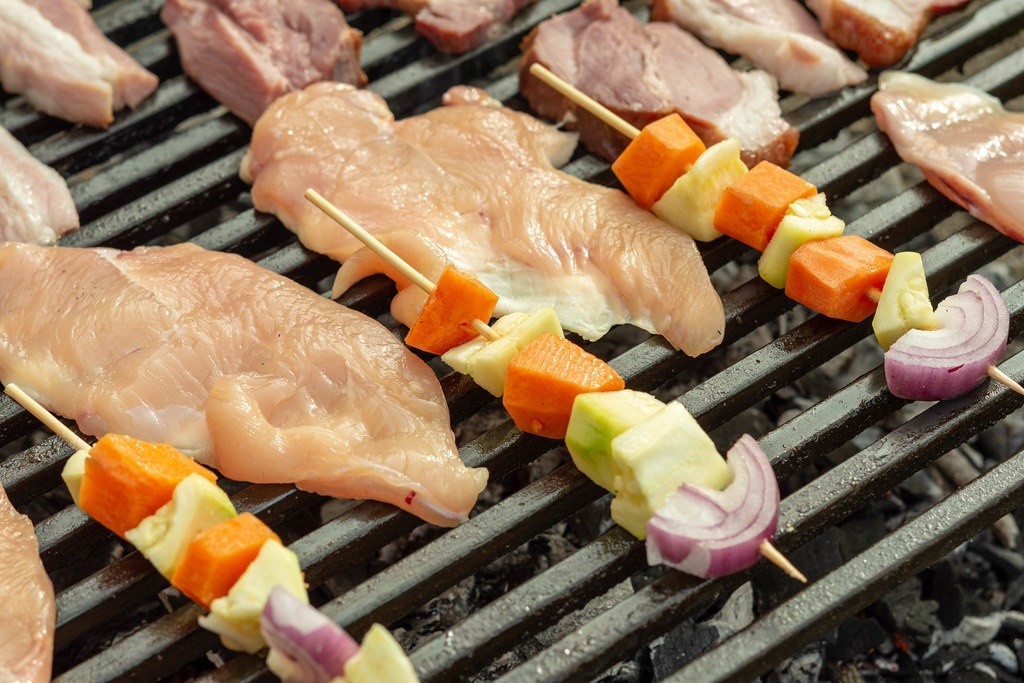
[[502, 332, 625, 438], [405, 262, 499, 355], [783, 234, 897, 321], [81, 433, 217, 535], [171, 513, 283, 609], [716, 159, 819, 251], [613, 113, 708, 205]]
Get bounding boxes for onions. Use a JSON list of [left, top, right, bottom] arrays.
[[260, 586, 361, 678], [884, 275, 1010, 398], [644, 434, 781, 576]]
